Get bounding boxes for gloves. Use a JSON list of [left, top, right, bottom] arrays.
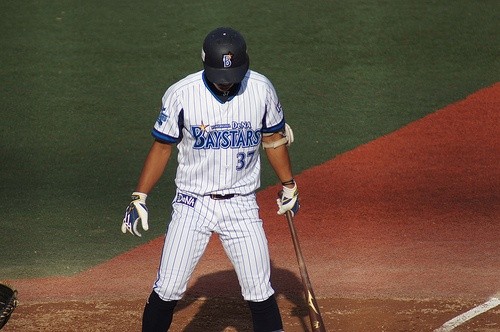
[[120, 192, 149, 238], [276, 181, 300, 218]]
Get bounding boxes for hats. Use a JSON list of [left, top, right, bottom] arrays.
[[201, 27, 250, 86]]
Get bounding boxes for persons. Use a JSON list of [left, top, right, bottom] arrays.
[[121, 27, 299, 332]]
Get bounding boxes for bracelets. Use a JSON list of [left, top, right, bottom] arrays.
[[282, 179, 295, 186]]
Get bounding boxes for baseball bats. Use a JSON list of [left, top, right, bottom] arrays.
[[278, 189, 326, 331]]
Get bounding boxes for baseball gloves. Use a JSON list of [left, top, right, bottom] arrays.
[[0, 283, 18, 330]]
